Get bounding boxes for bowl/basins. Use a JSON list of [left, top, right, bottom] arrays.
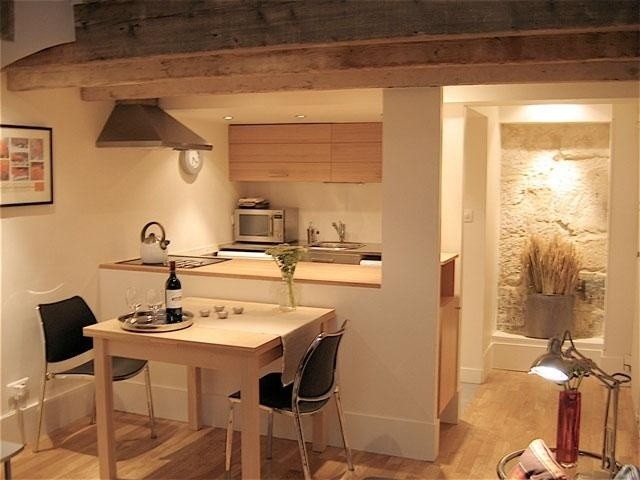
[[200, 306, 243, 318]]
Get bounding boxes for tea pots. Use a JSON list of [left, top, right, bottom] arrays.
[[138, 221, 170, 265]]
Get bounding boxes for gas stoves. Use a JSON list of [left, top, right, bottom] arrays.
[[117, 253, 231, 269]]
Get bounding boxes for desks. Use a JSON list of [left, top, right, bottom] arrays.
[[496, 446, 634, 480]]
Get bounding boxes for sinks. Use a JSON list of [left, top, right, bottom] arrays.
[[308, 242, 366, 252]]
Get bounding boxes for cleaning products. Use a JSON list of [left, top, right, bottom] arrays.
[[306, 220, 314, 244]]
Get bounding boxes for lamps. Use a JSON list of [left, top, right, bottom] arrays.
[[526, 328, 631, 474]]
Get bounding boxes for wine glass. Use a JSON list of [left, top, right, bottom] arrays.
[[146, 290, 163, 319], [125, 287, 144, 323]]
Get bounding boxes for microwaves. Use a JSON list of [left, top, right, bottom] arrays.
[[232, 209, 299, 244]]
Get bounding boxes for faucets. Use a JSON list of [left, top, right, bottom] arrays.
[[332, 220, 346, 242]]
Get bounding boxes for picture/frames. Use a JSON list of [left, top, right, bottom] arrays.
[[0, 123, 55, 207]]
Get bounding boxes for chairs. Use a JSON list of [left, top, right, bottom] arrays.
[[31, 295, 157, 459], [226, 317, 358, 478]]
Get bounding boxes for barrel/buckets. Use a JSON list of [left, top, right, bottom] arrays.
[[525, 293, 575, 339]]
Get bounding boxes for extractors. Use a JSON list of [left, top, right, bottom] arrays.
[[95, 100, 213, 150]]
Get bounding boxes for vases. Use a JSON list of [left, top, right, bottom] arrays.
[[524, 291, 578, 336]]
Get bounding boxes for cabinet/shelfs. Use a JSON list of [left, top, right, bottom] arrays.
[[228, 123, 382, 185]]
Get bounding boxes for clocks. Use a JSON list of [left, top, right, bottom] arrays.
[[180, 148, 203, 179]]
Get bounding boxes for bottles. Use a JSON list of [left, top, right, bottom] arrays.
[[163, 262, 183, 322]]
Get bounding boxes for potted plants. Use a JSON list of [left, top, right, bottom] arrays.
[[267, 241, 308, 314]]
